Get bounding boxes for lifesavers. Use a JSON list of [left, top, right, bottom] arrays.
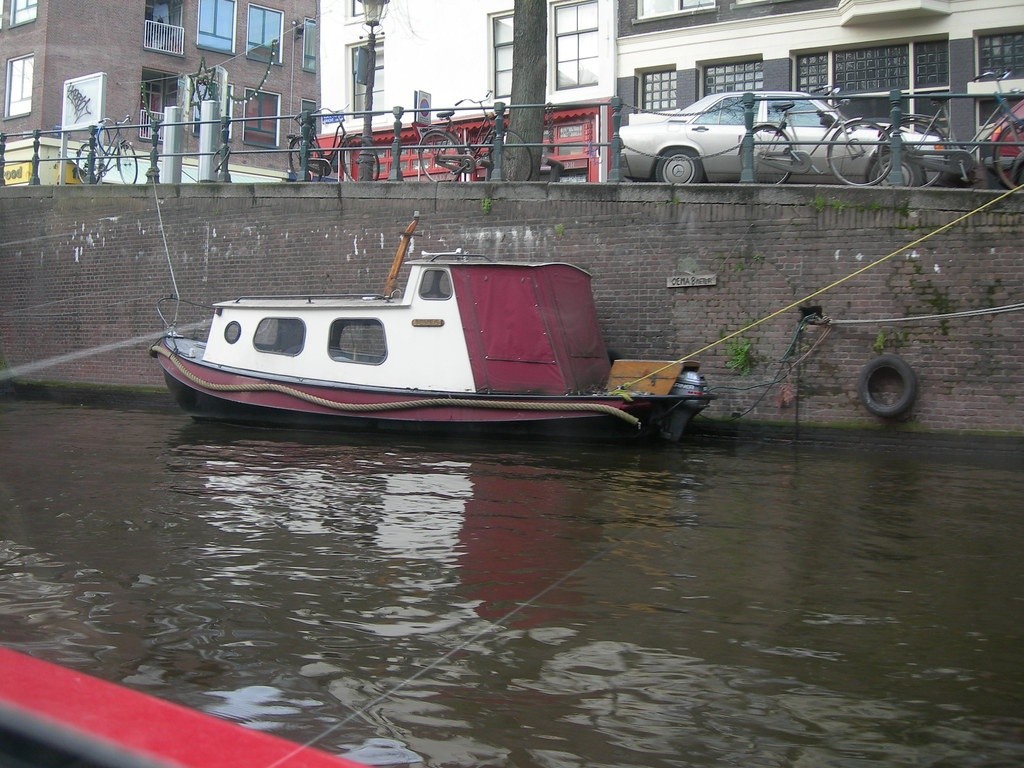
[[859, 353, 920, 420]]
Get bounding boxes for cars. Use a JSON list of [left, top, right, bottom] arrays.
[[985, 99, 1024, 188], [615, 92, 968, 190]]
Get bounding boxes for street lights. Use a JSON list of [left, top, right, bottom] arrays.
[[358, 0, 390, 183]]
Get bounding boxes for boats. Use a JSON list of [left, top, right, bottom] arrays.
[[150, 208, 723, 447]]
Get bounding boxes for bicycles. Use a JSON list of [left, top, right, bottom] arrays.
[[289, 103, 380, 183], [74, 115, 139, 185], [739, 85, 894, 188], [418, 88, 533, 183], [876, 68, 1023, 189]]
